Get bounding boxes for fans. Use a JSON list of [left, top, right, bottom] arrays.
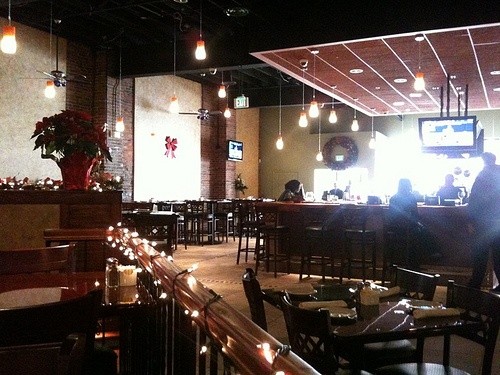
[[179, 74, 223, 118], [19, 15, 87, 82]]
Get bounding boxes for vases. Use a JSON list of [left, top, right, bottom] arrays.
[[236, 189, 240, 198], [56, 156, 96, 192]]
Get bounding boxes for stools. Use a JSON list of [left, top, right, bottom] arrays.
[[252, 205, 420, 288]]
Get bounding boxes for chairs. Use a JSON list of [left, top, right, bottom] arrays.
[[0, 286, 118, 375], [241, 262, 500, 375], [122, 196, 264, 251], [0, 239, 79, 274]]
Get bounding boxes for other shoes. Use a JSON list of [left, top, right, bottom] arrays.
[[490, 284, 500, 294]]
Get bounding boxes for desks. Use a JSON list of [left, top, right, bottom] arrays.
[[121, 210, 180, 257], [0, 271, 157, 375], [44, 227, 106, 246]]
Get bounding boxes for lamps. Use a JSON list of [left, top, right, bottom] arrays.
[[53, 78, 67, 87], [197, 113, 210, 121], [195, 0, 206, 60], [44, 0, 56, 99], [315, 103, 324, 162], [329, 84, 338, 124], [0, 0, 17, 54], [298, 67, 309, 127], [309, 48, 322, 118], [413, 33, 425, 91], [218, 70, 227, 99], [115, 31, 125, 133], [351, 98, 360, 132], [169, 18, 180, 115], [275, 74, 284, 151], [223, 86, 231, 118], [368, 107, 377, 150]]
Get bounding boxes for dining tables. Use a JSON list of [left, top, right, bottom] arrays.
[[261, 284, 483, 375]]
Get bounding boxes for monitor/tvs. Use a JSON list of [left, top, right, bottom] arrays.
[[419, 117, 476, 149], [228, 140, 243, 161]]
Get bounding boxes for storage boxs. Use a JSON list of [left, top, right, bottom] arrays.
[[356, 301, 380, 320]]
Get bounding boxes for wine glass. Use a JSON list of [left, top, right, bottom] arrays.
[[457, 191, 466, 204]]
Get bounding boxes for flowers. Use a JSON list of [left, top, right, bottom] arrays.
[[234, 172, 249, 195], [28, 108, 112, 163]]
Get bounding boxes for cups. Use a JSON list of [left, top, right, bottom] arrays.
[[327, 192, 350, 202], [305, 191, 314, 201]]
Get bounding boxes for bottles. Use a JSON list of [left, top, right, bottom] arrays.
[[355, 282, 364, 303], [383, 194, 391, 205], [105, 257, 120, 288], [364, 282, 371, 291]]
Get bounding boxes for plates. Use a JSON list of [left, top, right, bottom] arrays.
[[318, 307, 358, 323], [284, 288, 318, 296], [404, 299, 443, 312]]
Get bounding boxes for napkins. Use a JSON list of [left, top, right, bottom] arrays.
[[298, 300, 347, 310], [376, 285, 401, 298], [413, 307, 467, 321]]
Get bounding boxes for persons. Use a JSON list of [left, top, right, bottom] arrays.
[[470, 151, 500, 294], [278, 180, 305, 202], [387, 178, 420, 293], [436, 172, 462, 204]]
